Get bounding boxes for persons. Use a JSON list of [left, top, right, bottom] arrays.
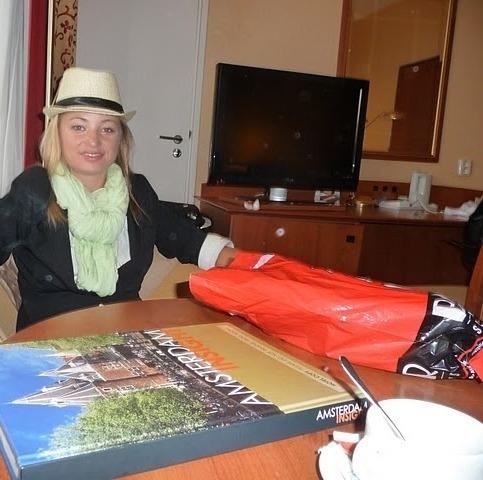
[[0, 68, 242, 334]]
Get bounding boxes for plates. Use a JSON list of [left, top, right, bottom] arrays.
[[316, 431, 360, 480]]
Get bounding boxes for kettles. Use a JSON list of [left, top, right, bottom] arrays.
[[408, 170, 433, 210]]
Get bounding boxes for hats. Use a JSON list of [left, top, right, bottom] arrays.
[[42, 67, 135, 120]]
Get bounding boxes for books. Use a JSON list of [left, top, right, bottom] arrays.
[[0, 322, 370, 480]]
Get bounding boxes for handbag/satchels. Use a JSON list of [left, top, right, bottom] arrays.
[[182, 250, 482, 382]]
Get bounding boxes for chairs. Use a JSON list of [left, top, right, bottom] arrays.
[[0, 254, 23, 341]]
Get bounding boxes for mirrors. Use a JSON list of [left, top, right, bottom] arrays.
[[335, 1, 456, 164]]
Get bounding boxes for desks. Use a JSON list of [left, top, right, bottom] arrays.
[[0, 285, 483, 479]]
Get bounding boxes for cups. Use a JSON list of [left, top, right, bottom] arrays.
[[362, 398, 483, 480]]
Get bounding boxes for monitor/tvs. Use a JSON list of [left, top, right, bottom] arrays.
[[206, 63, 370, 206]]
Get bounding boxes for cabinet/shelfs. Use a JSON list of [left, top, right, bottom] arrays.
[[193, 184, 471, 290]]
